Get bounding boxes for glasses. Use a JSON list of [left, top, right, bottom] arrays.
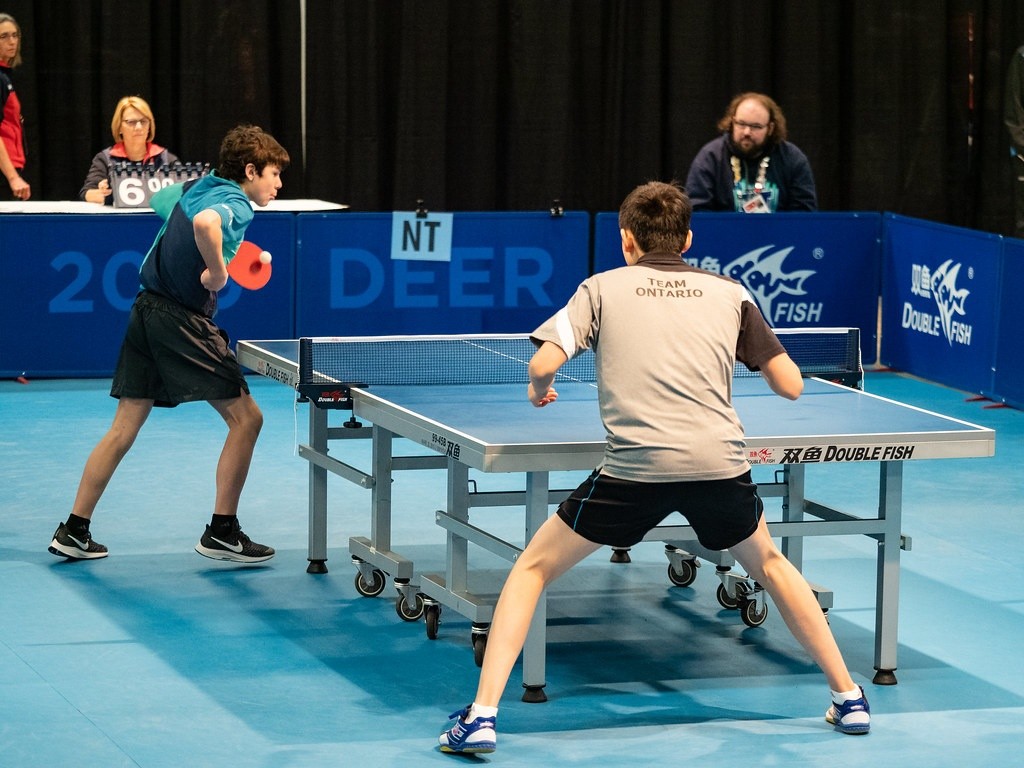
[[733, 118, 769, 131], [120, 119, 150, 126]]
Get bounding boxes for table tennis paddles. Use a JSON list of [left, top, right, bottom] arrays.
[[226, 240, 274, 291]]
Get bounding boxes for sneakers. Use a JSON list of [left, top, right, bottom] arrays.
[[48, 522, 109, 558], [439, 703, 496, 753], [195, 518, 275, 563], [826, 686, 871, 733]]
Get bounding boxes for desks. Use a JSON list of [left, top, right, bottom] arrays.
[[231, 333, 995, 705], [0, 197, 351, 217]]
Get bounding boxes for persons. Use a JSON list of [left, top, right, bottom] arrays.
[[0, 13, 31, 201], [684, 93, 819, 213], [439, 182, 870, 753], [49, 123, 289, 565], [78, 96, 182, 205]]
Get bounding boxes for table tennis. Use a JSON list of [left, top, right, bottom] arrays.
[[258, 250, 272, 264]]
